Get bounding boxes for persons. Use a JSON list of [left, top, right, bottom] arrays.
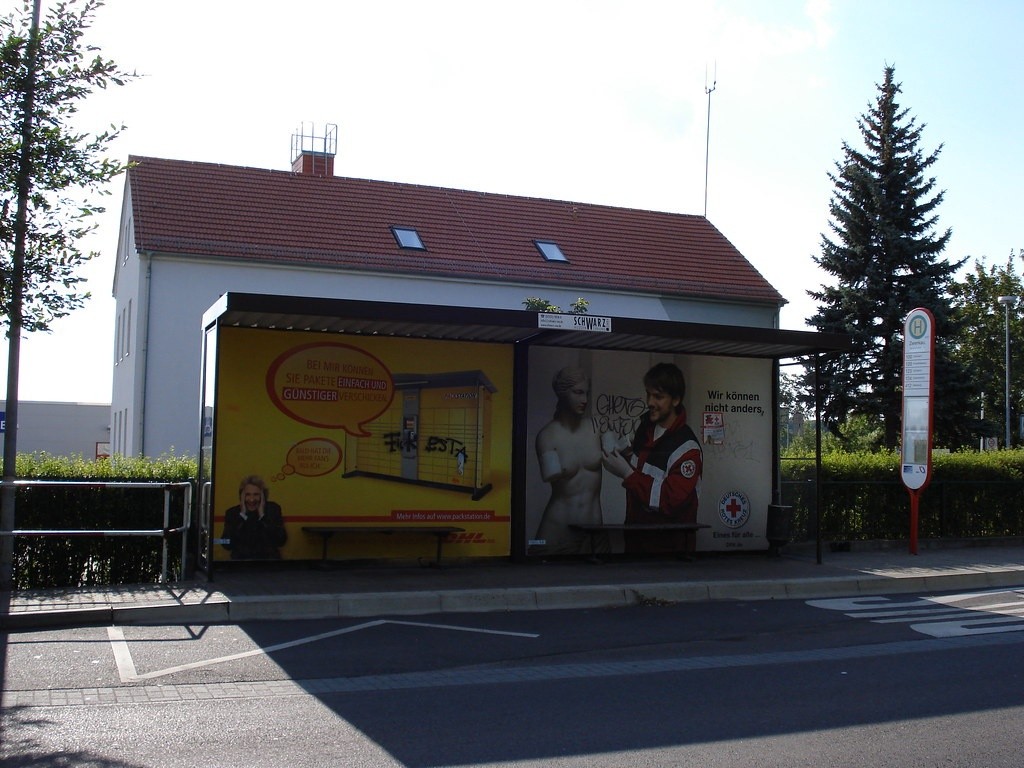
[[528, 366, 612, 554], [220, 475, 288, 559], [602, 363, 704, 554]]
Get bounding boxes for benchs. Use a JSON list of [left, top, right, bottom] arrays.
[[567, 524, 712, 564], [301, 526, 467, 569]]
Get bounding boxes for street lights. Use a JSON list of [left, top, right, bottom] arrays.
[[998, 296, 1016, 448]]
